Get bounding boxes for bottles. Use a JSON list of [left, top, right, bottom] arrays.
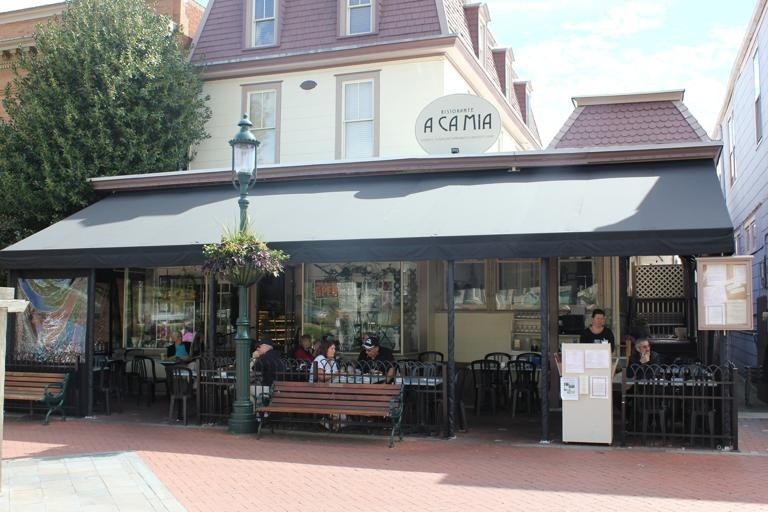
[[513, 333, 521, 349]]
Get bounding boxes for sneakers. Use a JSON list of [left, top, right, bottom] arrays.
[[319, 416, 349, 430]]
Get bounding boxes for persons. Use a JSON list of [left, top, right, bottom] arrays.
[[248, 332, 405, 436], [165, 329, 199, 391], [579, 308, 614, 355], [613, 337, 664, 433]]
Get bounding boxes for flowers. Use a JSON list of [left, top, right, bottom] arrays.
[[200, 214, 291, 279]]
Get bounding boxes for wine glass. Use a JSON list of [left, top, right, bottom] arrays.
[[674, 328, 687, 341], [513, 311, 541, 333], [130, 336, 140, 347]]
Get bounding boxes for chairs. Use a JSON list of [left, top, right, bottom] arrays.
[[5, 371, 70, 425]]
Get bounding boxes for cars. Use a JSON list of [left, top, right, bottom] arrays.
[[146, 307, 233, 348]]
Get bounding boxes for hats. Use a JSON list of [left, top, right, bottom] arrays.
[[256, 338, 274, 346], [361, 337, 380, 349]]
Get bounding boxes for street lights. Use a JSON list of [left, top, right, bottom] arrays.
[[226, 110, 263, 439]]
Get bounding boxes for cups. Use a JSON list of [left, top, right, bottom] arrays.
[[334, 368, 382, 381], [500, 361, 505, 369], [530, 343, 538, 352]]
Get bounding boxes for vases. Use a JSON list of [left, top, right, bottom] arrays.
[[226, 265, 263, 287]]
